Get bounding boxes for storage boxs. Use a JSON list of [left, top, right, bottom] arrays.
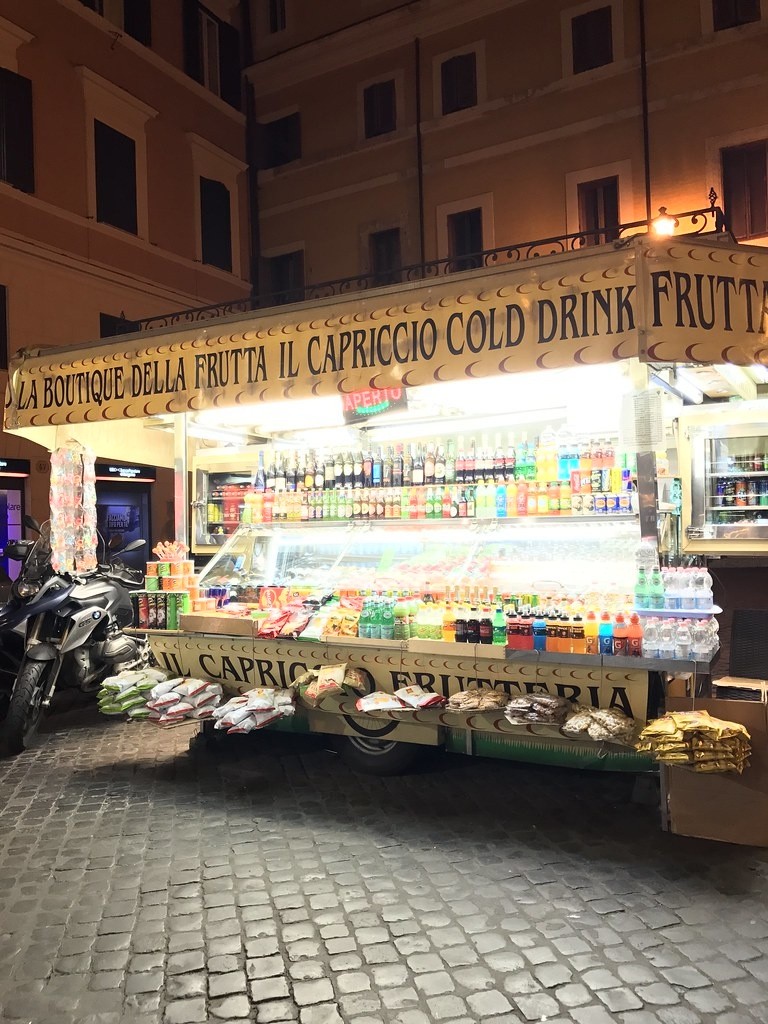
[[180, 610, 262, 637]]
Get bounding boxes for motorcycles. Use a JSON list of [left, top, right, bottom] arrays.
[[0, 512, 150, 756]]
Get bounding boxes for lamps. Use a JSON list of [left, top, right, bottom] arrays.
[[651, 207, 676, 235]]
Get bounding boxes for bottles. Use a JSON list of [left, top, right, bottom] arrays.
[[209, 432, 614, 520], [199, 564, 721, 661]]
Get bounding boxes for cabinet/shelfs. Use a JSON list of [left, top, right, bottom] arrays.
[[677, 398, 768, 557], [190, 415, 679, 564]]
